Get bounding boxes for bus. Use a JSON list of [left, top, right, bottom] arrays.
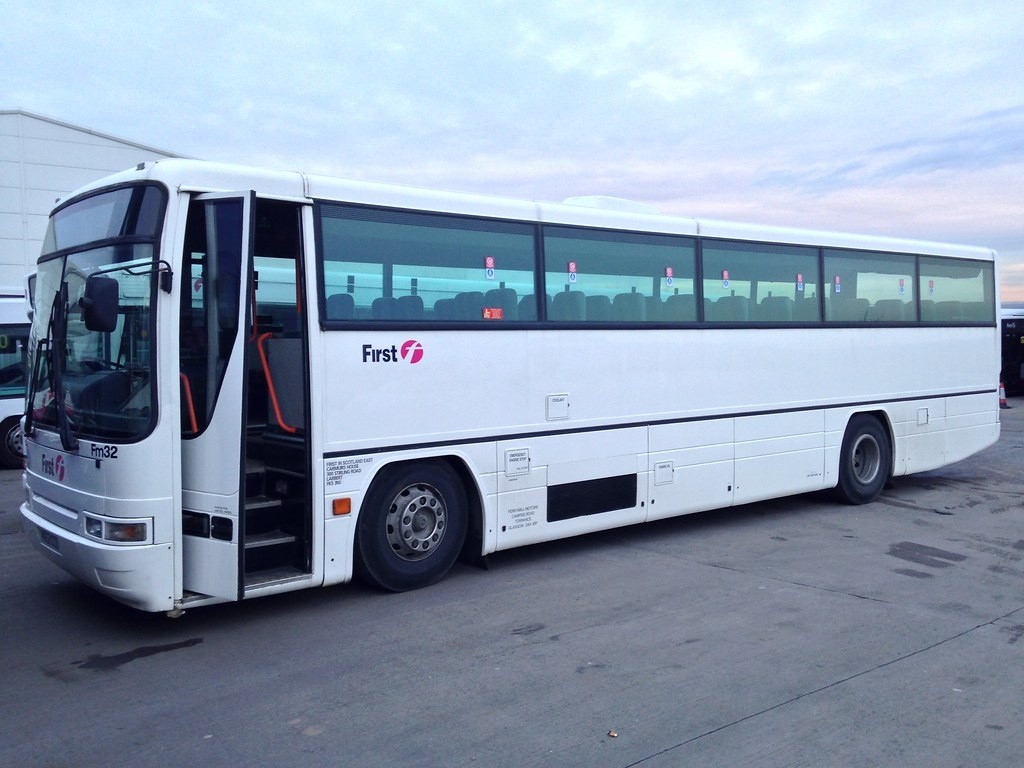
[[74, 255, 672, 374], [20, 159, 1004, 618], [0, 292, 41, 469]]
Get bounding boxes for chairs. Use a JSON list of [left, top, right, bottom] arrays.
[[326, 288, 986, 320]]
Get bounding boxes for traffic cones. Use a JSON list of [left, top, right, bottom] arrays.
[[999, 382, 1011, 409]]
